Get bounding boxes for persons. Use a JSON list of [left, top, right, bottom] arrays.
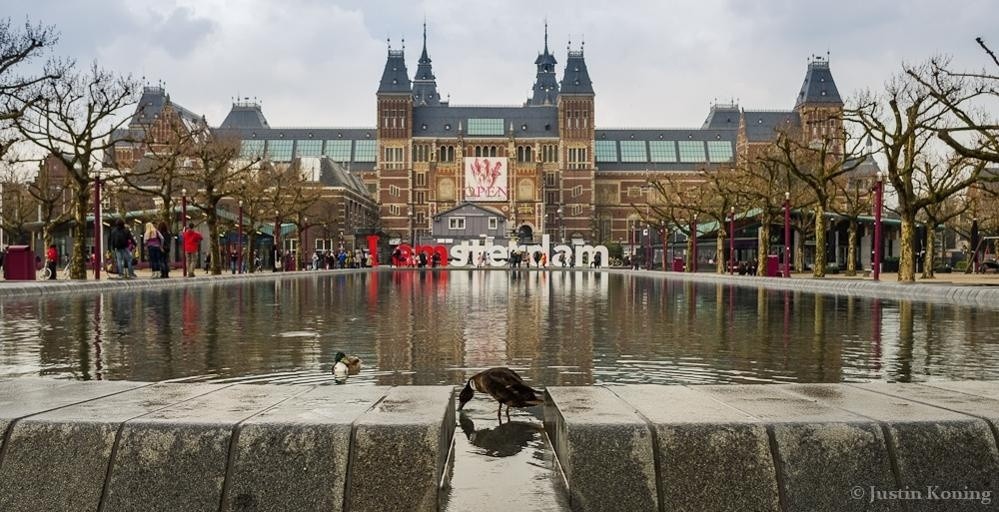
[[45, 219, 450, 283], [466, 249, 602, 269], [727, 257, 759, 274]]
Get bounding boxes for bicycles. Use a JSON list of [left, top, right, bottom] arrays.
[[60, 255, 74, 281], [38, 255, 54, 282]]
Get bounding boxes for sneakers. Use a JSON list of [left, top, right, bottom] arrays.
[[150, 271, 170, 279], [188, 271, 195, 278], [117, 272, 137, 279]]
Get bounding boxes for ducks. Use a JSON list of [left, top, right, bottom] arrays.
[[460, 411, 541, 457], [332, 352, 362, 375], [458, 368, 544, 415]]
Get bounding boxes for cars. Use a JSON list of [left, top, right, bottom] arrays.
[[925, 250, 976, 271]]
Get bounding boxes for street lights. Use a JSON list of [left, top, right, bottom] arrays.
[[302, 215, 309, 270], [630, 220, 668, 274], [340, 231, 345, 252], [782, 191, 795, 279], [691, 212, 700, 270], [181, 187, 192, 277], [79, 268, 369, 381], [406, 210, 415, 245], [237, 201, 242, 275], [90, 165, 101, 278], [322, 224, 329, 253], [726, 204, 736, 274], [873, 170, 883, 280], [273, 209, 282, 272], [556, 206, 563, 243], [622, 276, 886, 383]]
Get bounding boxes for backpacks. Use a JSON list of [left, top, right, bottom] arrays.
[[114, 228, 128, 249]]
